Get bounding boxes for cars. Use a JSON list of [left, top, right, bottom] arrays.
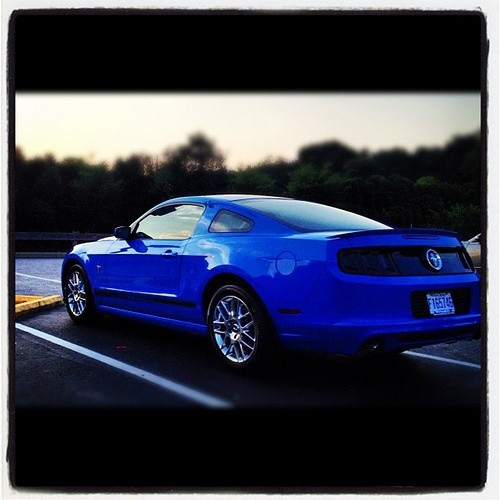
[[61, 194, 483, 372]]
